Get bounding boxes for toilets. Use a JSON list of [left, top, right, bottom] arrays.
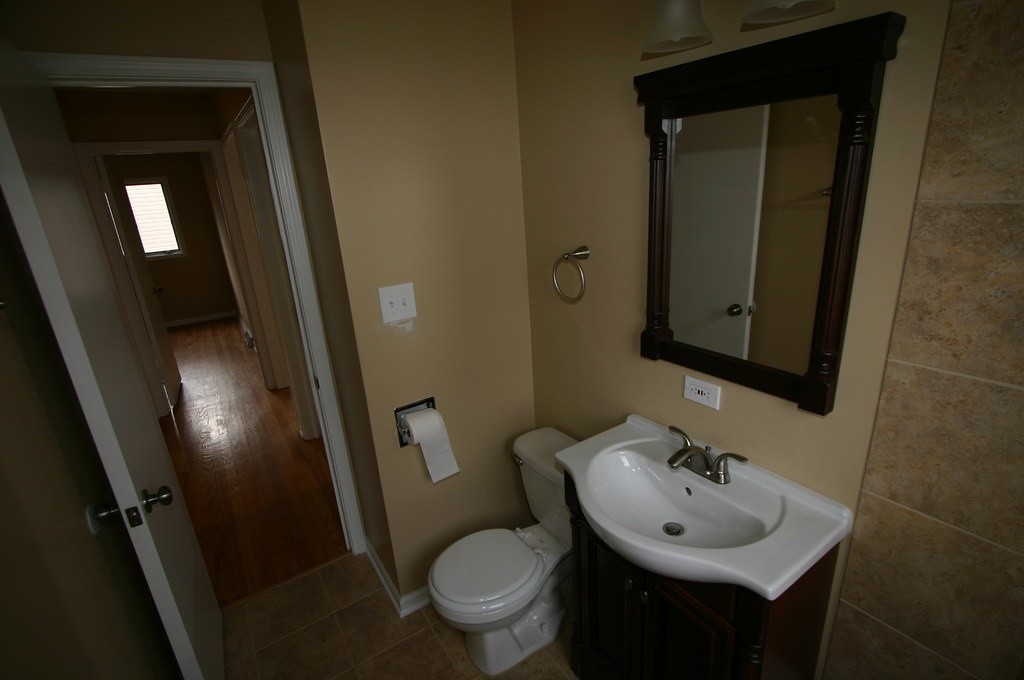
[[426, 425, 581, 677]]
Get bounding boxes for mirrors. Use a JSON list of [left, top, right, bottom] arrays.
[[633, 13, 906, 415]]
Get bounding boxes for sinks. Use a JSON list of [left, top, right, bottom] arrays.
[[554, 412, 854, 602]]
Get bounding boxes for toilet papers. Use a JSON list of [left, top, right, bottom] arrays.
[[400, 407, 461, 485]]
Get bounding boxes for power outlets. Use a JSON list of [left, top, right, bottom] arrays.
[[683, 375, 721, 411]]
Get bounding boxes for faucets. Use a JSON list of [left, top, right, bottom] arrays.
[[666, 425, 749, 486]]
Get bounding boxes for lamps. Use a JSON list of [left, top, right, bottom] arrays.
[[741, 0, 835, 23], [640, 0, 713, 53]]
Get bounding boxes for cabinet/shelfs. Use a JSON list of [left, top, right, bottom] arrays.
[[563, 471, 840, 680]]
